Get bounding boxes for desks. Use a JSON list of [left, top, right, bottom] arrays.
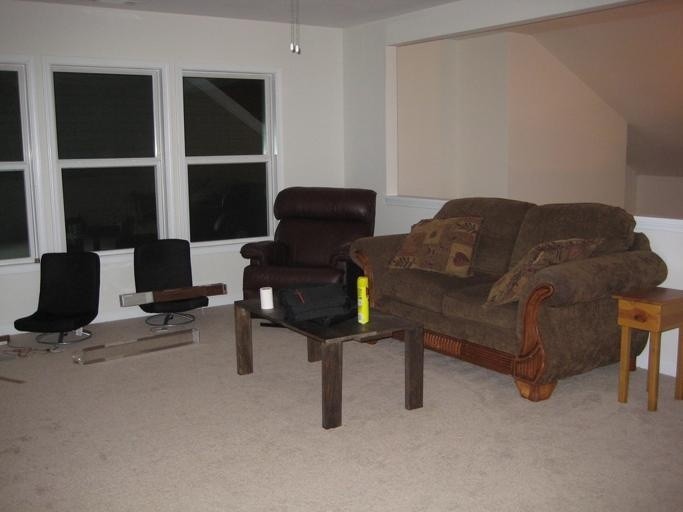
[[610, 287, 683, 412]]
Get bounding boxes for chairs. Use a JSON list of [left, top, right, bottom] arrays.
[[13, 238, 209, 346], [240, 186, 376, 319]]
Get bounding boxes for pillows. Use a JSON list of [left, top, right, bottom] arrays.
[[480, 238, 604, 311], [384, 216, 485, 279]]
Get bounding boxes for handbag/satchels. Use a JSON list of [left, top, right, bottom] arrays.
[[278, 284, 354, 328]]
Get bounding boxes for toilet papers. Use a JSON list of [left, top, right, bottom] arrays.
[[260, 287, 274, 310]]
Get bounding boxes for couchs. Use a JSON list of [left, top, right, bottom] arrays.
[[349, 197, 668, 402]]
